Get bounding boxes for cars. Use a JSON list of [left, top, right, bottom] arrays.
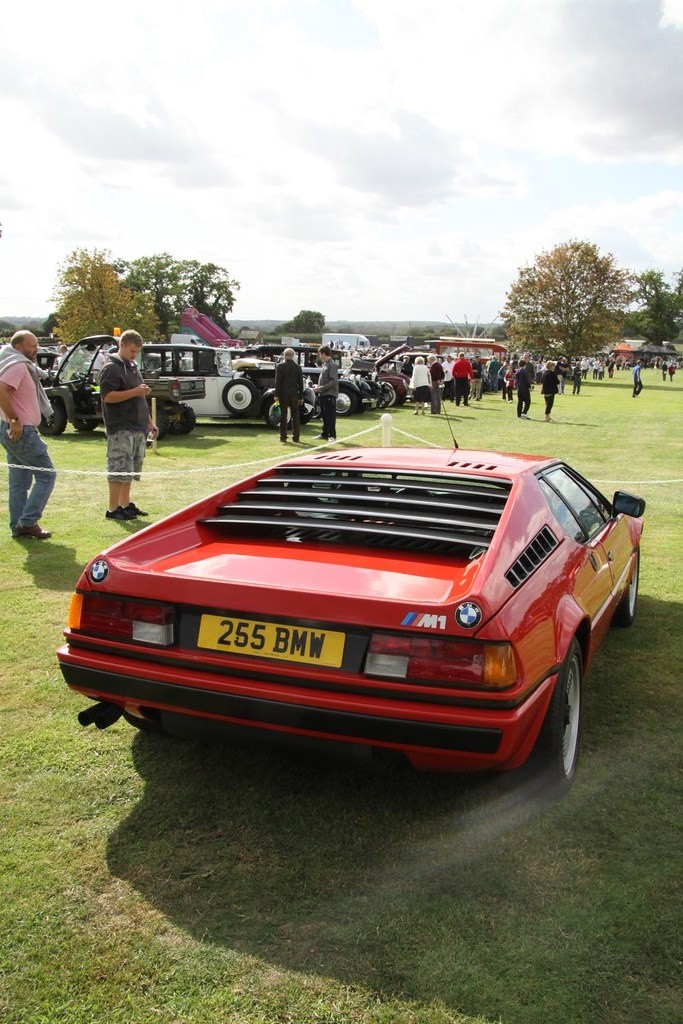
[[371, 343, 414, 407]]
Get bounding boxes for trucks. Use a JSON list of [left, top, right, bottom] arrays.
[[322, 333, 370, 349]]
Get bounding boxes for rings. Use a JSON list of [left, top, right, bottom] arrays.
[[153, 427, 158, 431]]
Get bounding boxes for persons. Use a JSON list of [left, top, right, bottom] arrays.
[[632, 359, 647, 398], [581, 355, 631, 380], [89, 350, 106, 371], [313, 346, 339, 442], [556, 357, 582, 395], [308, 355, 317, 367], [0, 329, 56, 537], [408, 356, 433, 415], [427, 355, 445, 414], [452, 352, 474, 407], [540, 361, 560, 422], [99, 329, 157, 520], [654, 359, 675, 382], [58, 345, 84, 372], [402, 355, 544, 404], [274, 348, 304, 443], [362, 346, 391, 358], [514, 360, 531, 420]]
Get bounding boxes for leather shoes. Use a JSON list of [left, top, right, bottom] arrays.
[[12, 523, 51, 538]]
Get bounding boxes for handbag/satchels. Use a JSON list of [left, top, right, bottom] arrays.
[[269, 400, 281, 417]]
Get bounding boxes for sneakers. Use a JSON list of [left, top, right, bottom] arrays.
[[124, 503, 148, 516], [106, 506, 136, 520]]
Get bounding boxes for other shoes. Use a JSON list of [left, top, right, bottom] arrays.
[[293, 437, 299, 442], [518, 413, 531, 420], [281, 438, 286, 442], [328, 435, 336, 442], [412, 411, 418, 415], [422, 412, 425, 415], [313, 434, 328, 439]]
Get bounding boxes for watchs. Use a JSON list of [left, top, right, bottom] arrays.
[[10, 417, 19, 424]]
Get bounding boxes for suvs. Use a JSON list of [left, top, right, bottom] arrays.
[[141, 344, 317, 430], [249, 345, 381, 415]]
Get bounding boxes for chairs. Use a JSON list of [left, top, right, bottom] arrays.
[[334, 483, 369, 522], [385, 487, 434, 529]]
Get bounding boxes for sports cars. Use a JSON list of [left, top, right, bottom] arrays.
[[56, 444, 644, 801]]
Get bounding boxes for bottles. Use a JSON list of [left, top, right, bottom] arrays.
[[276, 400, 281, 416], [146, 428, 156, 448]]
